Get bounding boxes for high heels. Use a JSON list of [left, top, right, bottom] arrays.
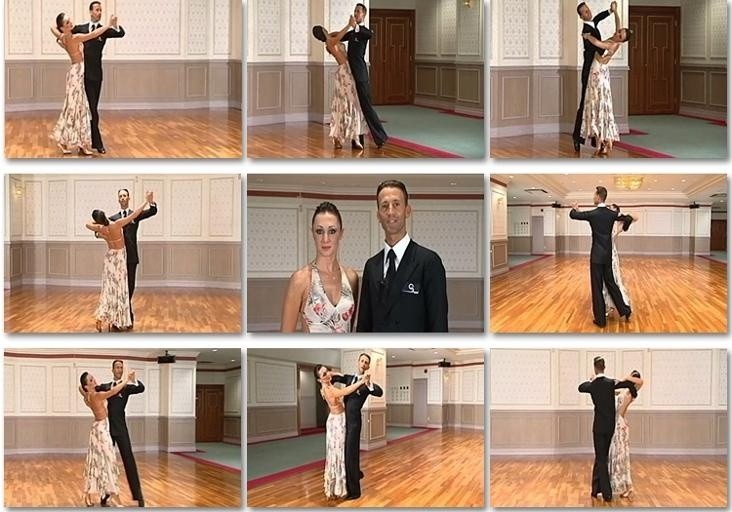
[[333, 136, 342, 149], [620, 486, 633, 498], [599, 140, 613, 154], [81, 491, 94, 506], [110, 498, 126, 508], [76, 142, 93, 155], [593, 137, 606, 156], [604, 307, 614, 317], [351, 137, 364, 150], [56, 142, 72, 154]]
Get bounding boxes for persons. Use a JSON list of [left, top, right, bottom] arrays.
[[319, 352, 384, 501], [572, 1, 616, 152], [322, 3, 390, 150], [355, 181, 449, 332], [280, 202, 361, 333], [314, 363, 369, 502], [78, 358, 147, 508], [582, 2, 635, 155], [76, 369, 135, 507], [94, 188, 159, 329], [569, 187, 638, 326], [570, 201, 638, 319], [49, 10, 117, 156], [84, 189, 149, 332], [577, 355, 638, 504], [586, 370, 645, 500], [50, 1, 128, 155], [312, 15, 366, 151]]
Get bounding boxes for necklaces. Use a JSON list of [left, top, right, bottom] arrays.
[[319, 268, 341, 282]]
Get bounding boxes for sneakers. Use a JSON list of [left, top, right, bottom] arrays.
[[96, 319, 101, 332]]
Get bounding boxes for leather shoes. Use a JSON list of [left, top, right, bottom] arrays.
[[127, 321, 133, 329], [378, 142, 384, 148], [139, 499, 144, 507], [573, 139, 580, 152], [101, 494, 110, 506], [97, 147, 105, 154]]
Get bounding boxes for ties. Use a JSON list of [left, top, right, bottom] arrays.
[[123, 210, 126, 217], [92, 24, 95, 31], [113, 382, 116, 386], [383, 249, 397, 297], [353, 376, 358, 384]]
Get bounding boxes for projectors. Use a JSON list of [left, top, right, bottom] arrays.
[[158, 355, 176, 364], [690, 204, 699, 209], [552, 203, 562, 208], [438, 361, 451, 367]]
